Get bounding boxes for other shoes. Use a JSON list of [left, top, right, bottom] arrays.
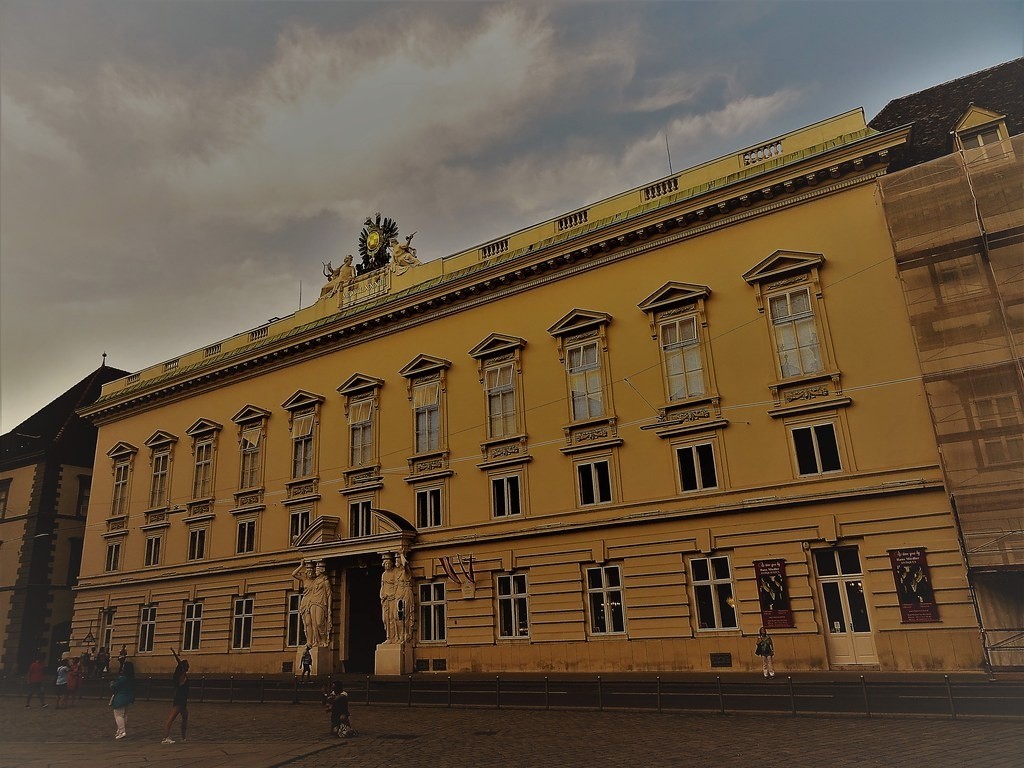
[[765, 675, 769, 679], [162, 737, 175, 744], [772, 675, 775, 678], [114, 730, 126, 739], [299, 680, 304, 683], [25, 704, 32, 709], [181, 739, 190, 744], [308, 680, 314, 683], [41, 704, 49, 708]]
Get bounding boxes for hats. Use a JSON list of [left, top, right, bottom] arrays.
[[306, 644, 312, 649], [341, 692, 348, 696]]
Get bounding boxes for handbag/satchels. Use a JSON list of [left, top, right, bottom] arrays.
[[337, 723, 360, 738], [309, 659, 312, 666], [755, 645, 762, 656]]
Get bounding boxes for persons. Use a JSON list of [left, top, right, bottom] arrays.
[[761, 576, 783, 610], [299, 644, 313, 684], [163, 660, 195, 745], [332, 254, 355, 291], [55, 658, 81, 709], [899, 563, 929, 603], [756, 626, 775, 681], [379, 548, 418, 644], [292, 559, 332, 645], [389, 237, 420, 264], [85, 645, 111, 674], [109, 663, 138, 738], [118, 645, 128, 671], [24, 657, 48, 708], [323, 683, 352, 733]]
